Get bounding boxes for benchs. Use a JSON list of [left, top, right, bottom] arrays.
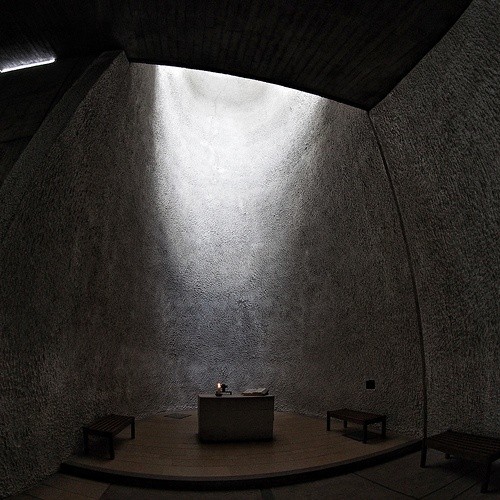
[[326, 408, 387, 445], [81, 413, 136, 460], [413, 430, 500, 494]]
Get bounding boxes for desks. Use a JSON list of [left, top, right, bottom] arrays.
[[196, 393, 276, 443]]
